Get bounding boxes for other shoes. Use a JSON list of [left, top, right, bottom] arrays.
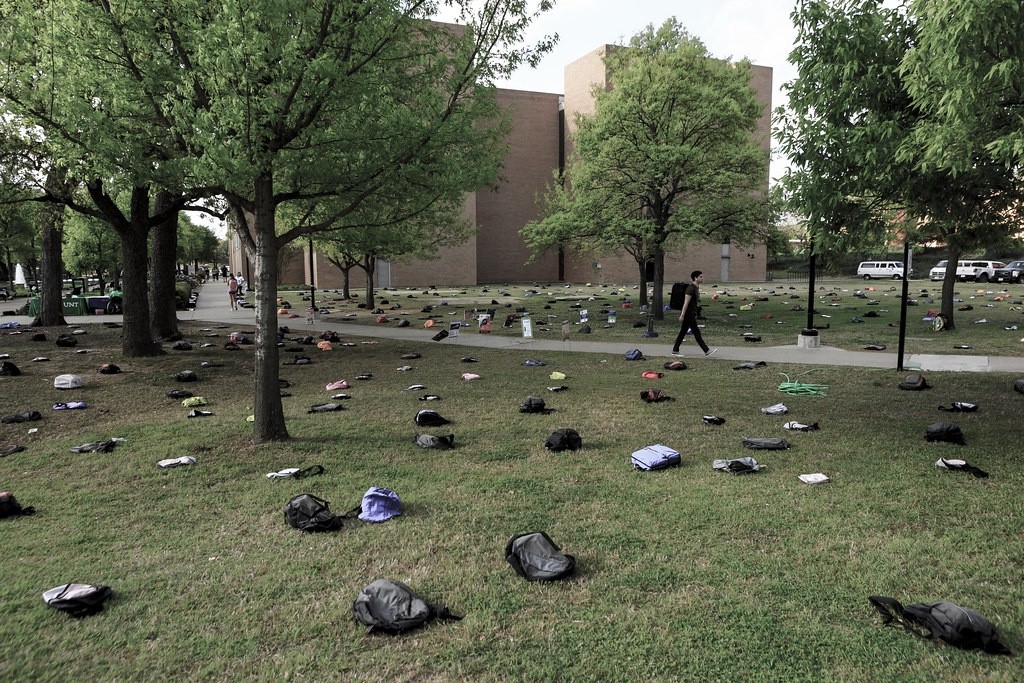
[[670, 351, 685, 358], [705, 348, 718, 358]]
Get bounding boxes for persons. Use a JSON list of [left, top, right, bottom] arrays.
[[671, 272, 719, 358], [204, 264, 244, 300], [227, 273, 238, 311]]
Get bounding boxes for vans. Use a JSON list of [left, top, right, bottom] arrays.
[[929, 259, 972, 282], [857, 259, 913, 280]]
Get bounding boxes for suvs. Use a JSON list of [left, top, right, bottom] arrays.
[[993, 261, 1024, 284]]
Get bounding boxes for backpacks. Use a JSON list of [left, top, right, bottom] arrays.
[[667, 282, 695, 311], [41, 582, 113, 616], [352, 578, 463, 635], [898, 373, 932, 390], [281, 493, 362, 532], [925, 422, 965, 445], [505, 531, 573, 583], [629, 444, 682, 471]]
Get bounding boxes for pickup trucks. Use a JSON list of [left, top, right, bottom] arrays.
[[956, 260, 1008, 283]]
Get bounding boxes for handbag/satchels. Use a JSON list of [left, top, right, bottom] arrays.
[[741, 436, 790, 450], [784, 421, 819, 433], [1, 282, 1024, 466], [543, 428, 581, 450], [414, 410, 450, 427], [869, 595, 1012, 654], [266, 464, 325, 481], [357, 486, 404, 521], [937, 456, 989, 479], [713, 456, 763, 474], [155, 455, 197, 468], [519, 396, 552, 416], [414, 433, 455, 451], [938, 401, 978, 412], [0, 492, 36, 517]]
